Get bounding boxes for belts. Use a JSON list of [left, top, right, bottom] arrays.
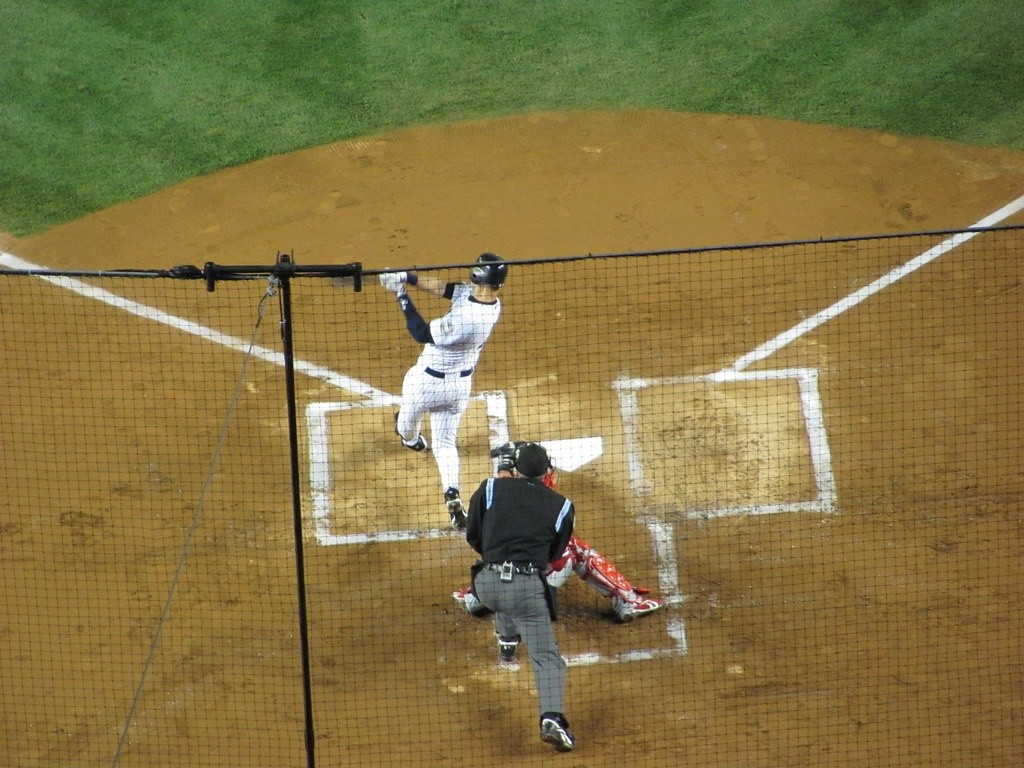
[[485, 562, 539, 574], [425, 367, 473, 378]]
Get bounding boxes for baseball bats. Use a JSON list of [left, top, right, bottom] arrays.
[[328, 274, 380, 286]]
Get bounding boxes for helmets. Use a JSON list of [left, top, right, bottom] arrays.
[[470, 253, 509, 288]]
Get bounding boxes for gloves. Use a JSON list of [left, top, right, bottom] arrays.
[[380, 271, 409, 298], [496, 440, 527, 471]]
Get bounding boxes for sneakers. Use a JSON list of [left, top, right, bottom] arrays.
[[452, 590, 483, 613], [444, 488, 469, 532], [394, 411, 428, 452], [497, 634, 522, 660], [619, 598, 664, 621], [539, 712, 574, 752]]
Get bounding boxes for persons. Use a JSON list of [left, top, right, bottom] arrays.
[[468, 444, 577, 751], [379, 253, 509, 529], [458, 441, 667, 623]]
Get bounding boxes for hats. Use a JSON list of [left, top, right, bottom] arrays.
[[512, 443, 550, 477]]
